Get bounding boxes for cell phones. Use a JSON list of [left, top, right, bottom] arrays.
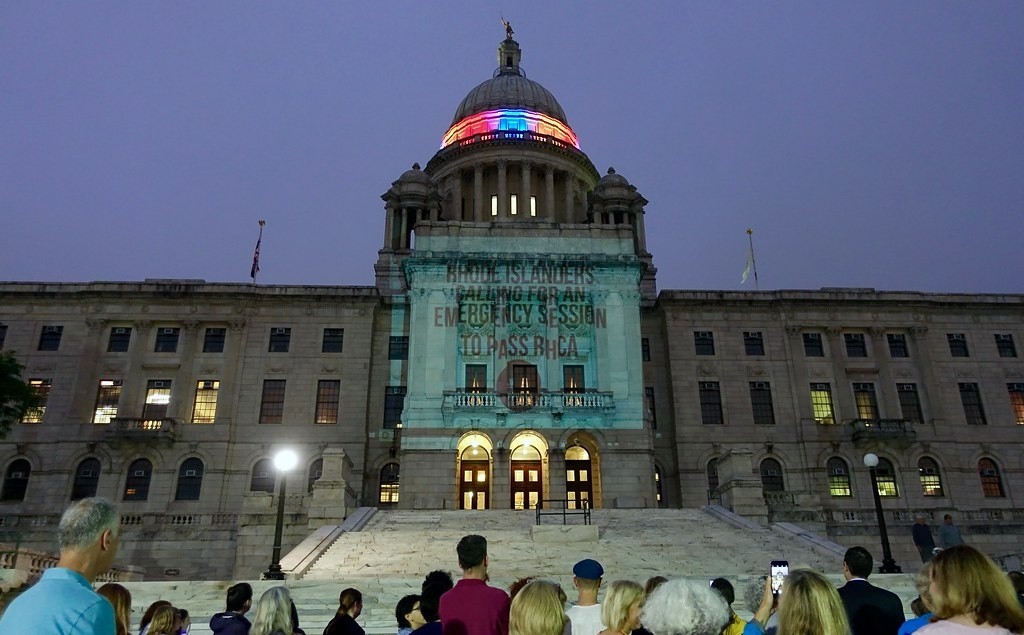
[[769, 560, 789, 608], [709, 580, 714, 586]]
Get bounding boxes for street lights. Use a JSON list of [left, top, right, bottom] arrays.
[[258, 447, 294, 580], [863, 452, 907, 574]]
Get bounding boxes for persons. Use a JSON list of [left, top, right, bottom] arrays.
[[396, 534, 607, 635], [209, 582, 307, 635], [0, 499, 135, 635], [502, 20, 514, 39], [940, 514, 966, 551], [323, 588, 366, 635], [139, 600, 192, 635], [911, 513, 935, 562], [598, 547, 906, 635], [899, 543, 1024, 635]]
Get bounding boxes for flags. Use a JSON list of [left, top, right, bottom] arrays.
[[251, 239, 260, 278]]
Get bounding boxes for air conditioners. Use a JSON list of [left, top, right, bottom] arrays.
[[154, 381, 164, 387], [858, 382, 1023, 392], [767, 470, 778, 475], [113, 379, 121, 386], [314, 469, 322, 476], [11, 471, 23, 479], [815, 383, 825, 391], [924, 467, 936, 475], [42, 378, 51, 385], [41, 325, 286, 335], [82, 469, 93, 477], [134, 470, 145, 477], [259, 471, 268, 479], [204, 382, 214, 388], [186, 469, 195, 477], [698, 331, 1012, 340], [705, 382, 715, 389], [984, 468, 996, 477], [834, 468, 845, 474], [755, 381, 765, 389]]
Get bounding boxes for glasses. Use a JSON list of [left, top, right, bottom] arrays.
[[129, 609, 134, 615]]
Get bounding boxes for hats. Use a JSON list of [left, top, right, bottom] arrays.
[[573, 559, 604, 579]]
